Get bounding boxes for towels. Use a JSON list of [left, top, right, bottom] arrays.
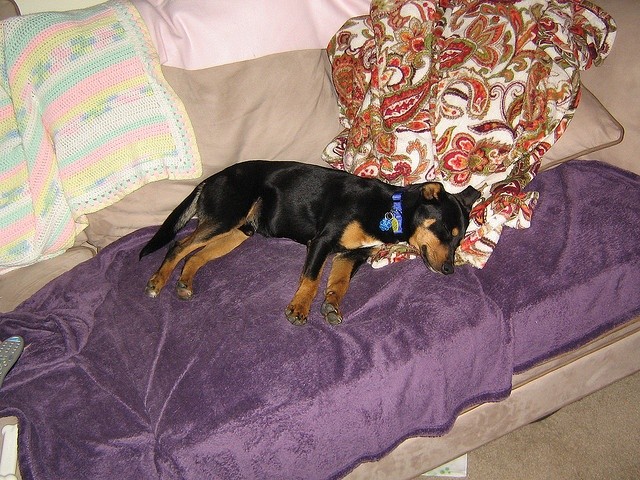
[[1, 158, 640, 480]]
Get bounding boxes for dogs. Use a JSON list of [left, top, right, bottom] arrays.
[[138, 158, 482, 327]]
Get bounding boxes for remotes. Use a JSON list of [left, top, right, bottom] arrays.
[[0, 335, 24, 393]]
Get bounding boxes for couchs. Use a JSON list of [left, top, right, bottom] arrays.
[[0, 0, 640, 480]]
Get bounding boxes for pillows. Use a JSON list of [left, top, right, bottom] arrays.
[[41, 48, 624, 258]]
